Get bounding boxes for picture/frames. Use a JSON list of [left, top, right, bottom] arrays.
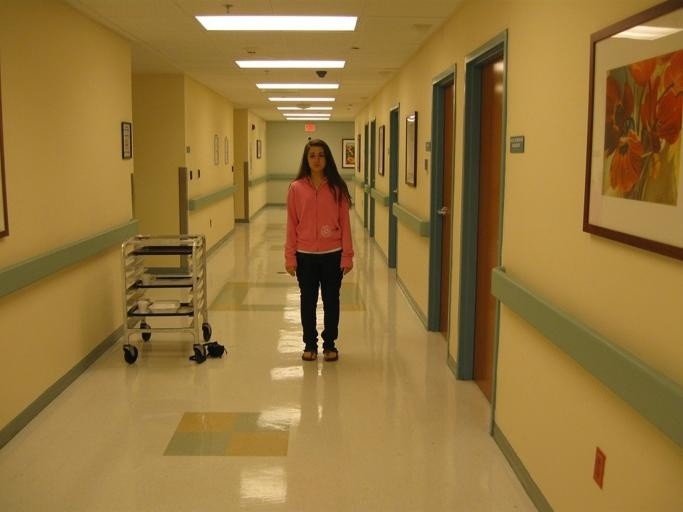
[[405, 111, 418, 188], [378, 125, 385, 176], [582, 1, 683, 260], [256, 139, 261, 158], [342, 138, 356, 168]]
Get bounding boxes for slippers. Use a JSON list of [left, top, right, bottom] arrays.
[[324, 351, 338, 361], [302, 350, 317, 361]]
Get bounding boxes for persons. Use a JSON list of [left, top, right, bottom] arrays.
[[282, 138, 356, 361]]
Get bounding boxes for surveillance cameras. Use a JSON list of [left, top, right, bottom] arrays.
[[316, 71, 327, 78]]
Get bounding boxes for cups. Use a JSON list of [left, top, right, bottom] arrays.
[[136, 300, 148, 313]]
